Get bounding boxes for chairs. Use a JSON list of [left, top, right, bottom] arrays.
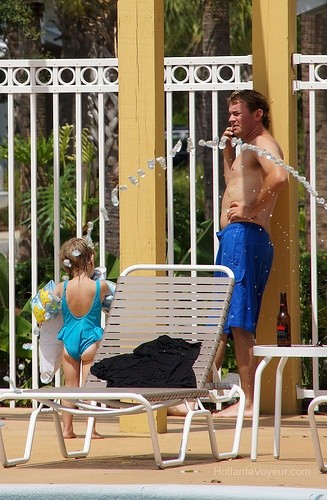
[[0, 264, 247, 471]]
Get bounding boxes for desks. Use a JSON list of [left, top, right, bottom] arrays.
[[251, 345, 327, 462]]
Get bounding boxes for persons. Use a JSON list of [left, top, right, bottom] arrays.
[[53, 238, 112, 439], [166, 89, 287, 417]]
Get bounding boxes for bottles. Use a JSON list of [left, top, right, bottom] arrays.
[[276, 293, 291, 346]]
